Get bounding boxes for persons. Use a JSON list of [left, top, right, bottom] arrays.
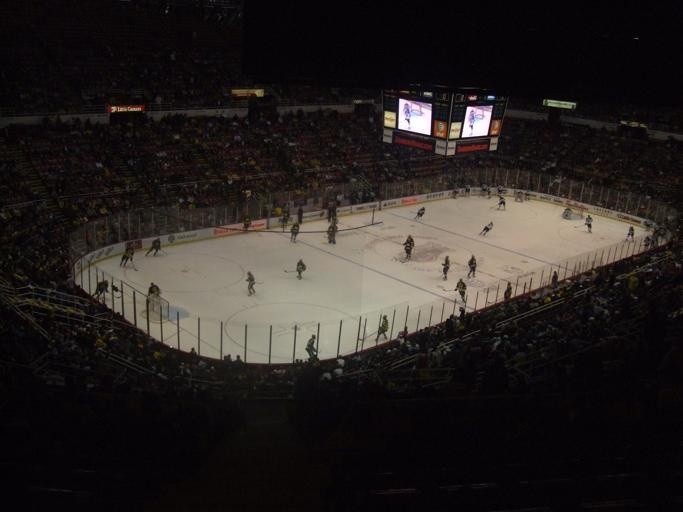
[[0, 83, 683, 512]]
